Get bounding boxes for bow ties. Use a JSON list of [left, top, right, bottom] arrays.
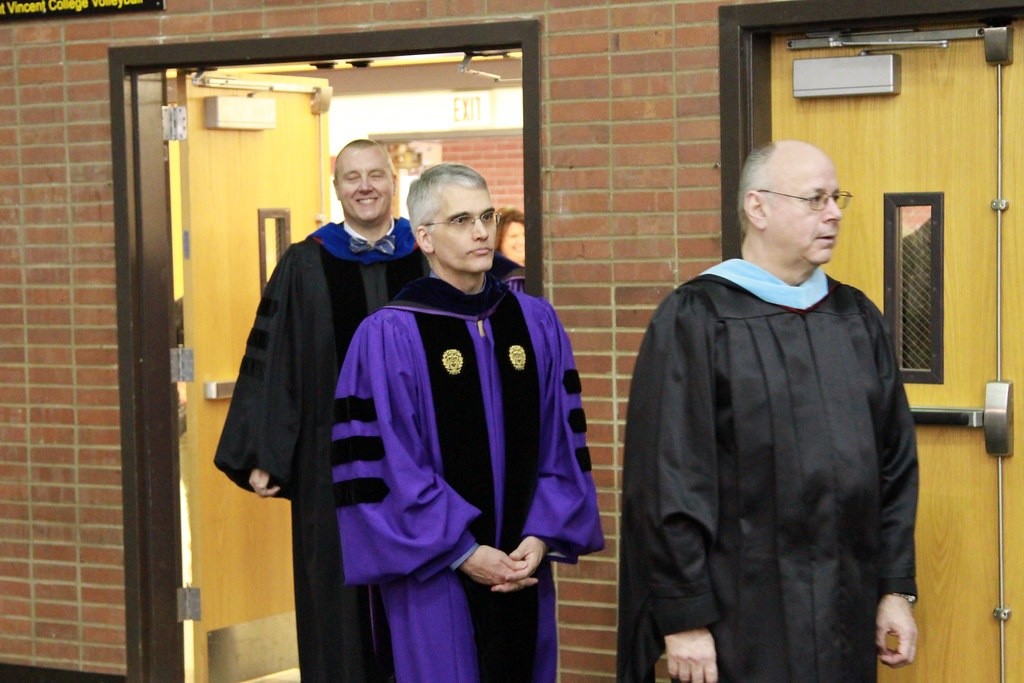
[[349, 235, 396, 255]]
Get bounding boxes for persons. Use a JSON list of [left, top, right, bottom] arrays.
[[212, 131, 427, 683], [493, 206, 528, 266], [615, 140, 919, 683], [330, 161, 606, 683]]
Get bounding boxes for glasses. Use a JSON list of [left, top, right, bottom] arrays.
[[756, 189, 853, 211], [424, 211, 503, 228]]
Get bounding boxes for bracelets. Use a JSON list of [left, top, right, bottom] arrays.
[[892, 592, 915, 603]]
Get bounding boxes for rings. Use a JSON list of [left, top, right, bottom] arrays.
[[519, 579, 524, 588]]
